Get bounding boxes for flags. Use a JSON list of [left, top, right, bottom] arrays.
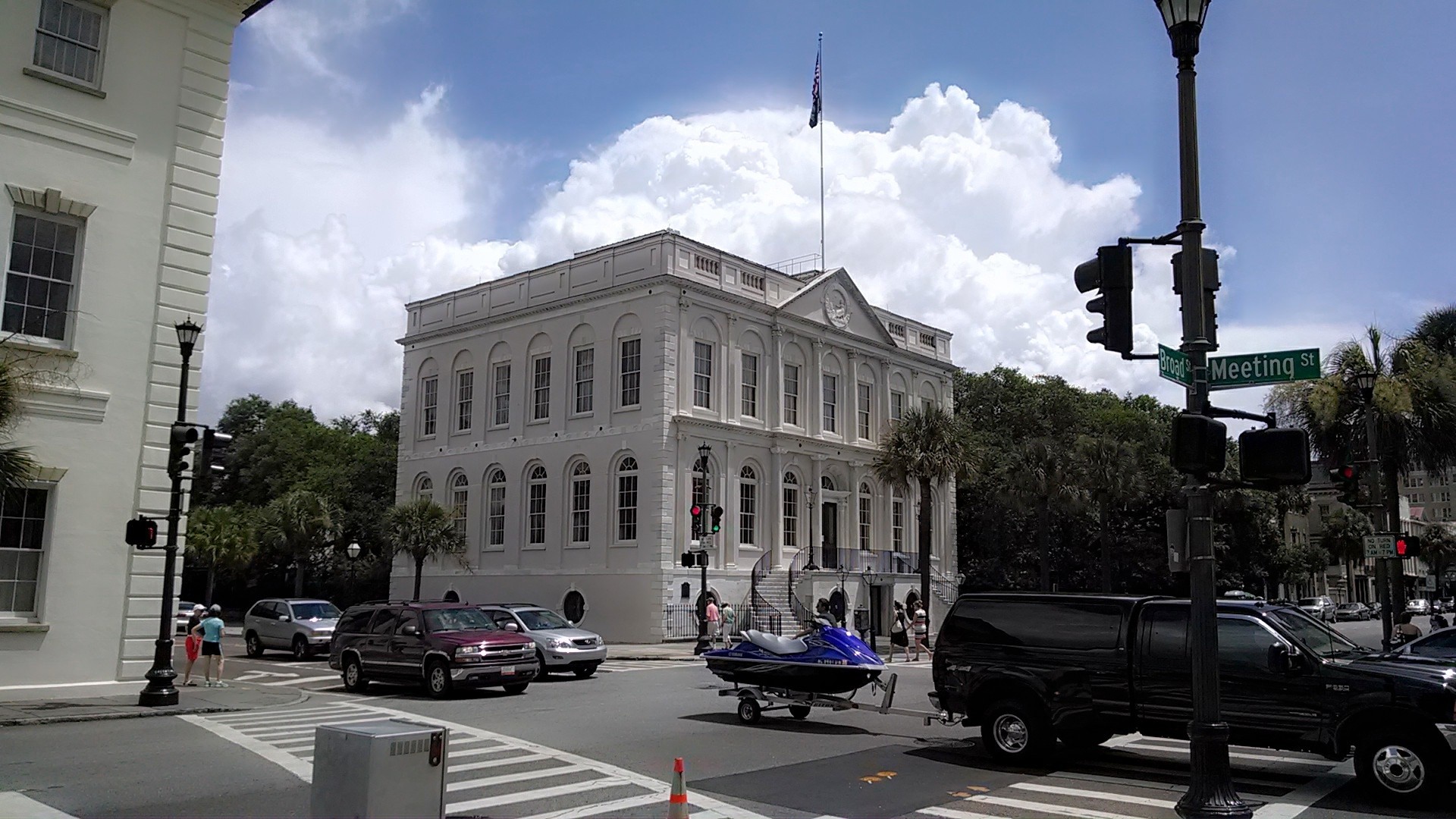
[[809, 52, 821, 128]]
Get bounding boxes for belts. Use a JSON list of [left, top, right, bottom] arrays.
[[187, 635, 193, 637], [708, 620, 718, 621]]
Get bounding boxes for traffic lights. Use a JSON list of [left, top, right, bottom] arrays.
[[689, 504, 702, 534], [1330, 467, 1350, 504], [165, 426, 199, 476], [1072, 243, 1134, 357], [1394, 536, 1420, 559], [198, 428, 233, 484], [711, 505, 724, 533], [136, 519, 158, 548], [1337, 464, 1360, 498]]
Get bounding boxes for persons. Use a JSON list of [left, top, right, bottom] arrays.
[[191, 604, 229, 688], [1389, 611, 1423, 652], [884, 600, 915, 663], [705, 597, 720, 651], [1427, 605, 1450, 634], [788, 598, 838, 640], [906, 599, 932, 662], [720, 601, 736, 650], [183, 604, 207, 686], [831, 604, 842, 629]]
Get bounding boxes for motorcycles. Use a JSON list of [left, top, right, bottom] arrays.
[[697, 609, 889, 695]]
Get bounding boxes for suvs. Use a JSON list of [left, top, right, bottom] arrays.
[[1297, 596, 1337, 624], [328, 599, 540, 700], [472, 601, 608, 681]]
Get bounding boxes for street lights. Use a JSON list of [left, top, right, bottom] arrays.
[[833, 564, 851, 629], [1167, 0, 1253, 819], [138, 313, 206, 705], [692, 441, 712, 656], [801, 487, 820, 572], [861, 566, 878, 653], [1355, 371, 1394, 653], [346, 537, 362, 606]]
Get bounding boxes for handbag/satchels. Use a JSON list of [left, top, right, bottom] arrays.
[[1390, 624, 1406, 648], [891, 610, 903, 633]]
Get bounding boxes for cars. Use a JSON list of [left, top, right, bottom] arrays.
[[238, 599, 345, 660], [1335, 594, 1455, 623], [176, 601, 203, 633]]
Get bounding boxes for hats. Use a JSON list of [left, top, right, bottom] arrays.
[[210, 604, 221, 611], [193, 604, 207, 612], [721, 601, 728, 608], [706, 597, 714, 601]]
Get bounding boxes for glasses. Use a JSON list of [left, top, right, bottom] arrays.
[[199, 610, 204, 613]]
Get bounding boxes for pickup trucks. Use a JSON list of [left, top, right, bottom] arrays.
[[923, 591, 1456, 809]]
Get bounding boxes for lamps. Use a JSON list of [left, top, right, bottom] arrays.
[[854, 603, 870, 634]]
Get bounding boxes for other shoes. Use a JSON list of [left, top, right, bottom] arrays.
[[214, 680, 229, 688], [203, 680, 213, 687], [183, 680, 198, 687], [729, 643, 733, 648], [722, 648, 729, 650], [884, 657, 892, 662], [904, 659, 910, 662], [912, 658, 919, 661]]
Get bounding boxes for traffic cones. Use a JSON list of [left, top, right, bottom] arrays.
[[668, 756, 690, 818]]
